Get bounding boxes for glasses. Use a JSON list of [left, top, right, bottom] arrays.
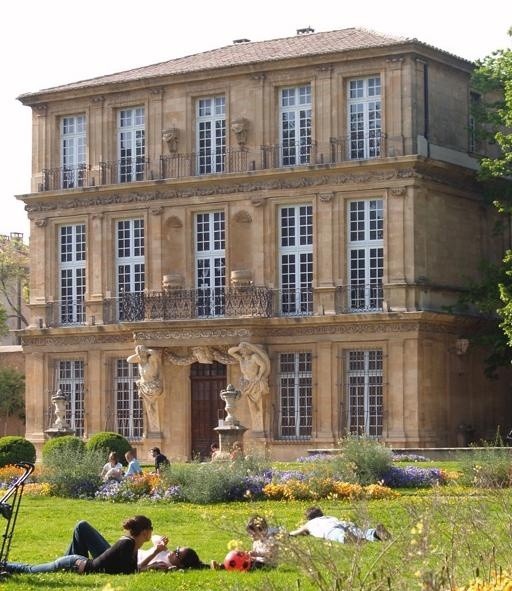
[[175, 546, 183, 562]]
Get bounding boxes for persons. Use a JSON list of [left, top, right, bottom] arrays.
[[6, 516, 167, 575], [228, 441, 244, 462], [212, 516, 278, 572], [228, 342, 266, 403], [101, 452, 122, 476], [210, 442, 226, 462], [66, 521, 199, 571], [103, 451, 143, 483], [127, 345, 162, 397], [289, 507, 392, 545], [150, 447, 170, 474]]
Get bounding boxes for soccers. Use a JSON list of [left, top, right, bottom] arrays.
[[223, 549, 251, 573]]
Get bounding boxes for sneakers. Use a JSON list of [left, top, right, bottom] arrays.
[[343, 522, 393, 546]]
